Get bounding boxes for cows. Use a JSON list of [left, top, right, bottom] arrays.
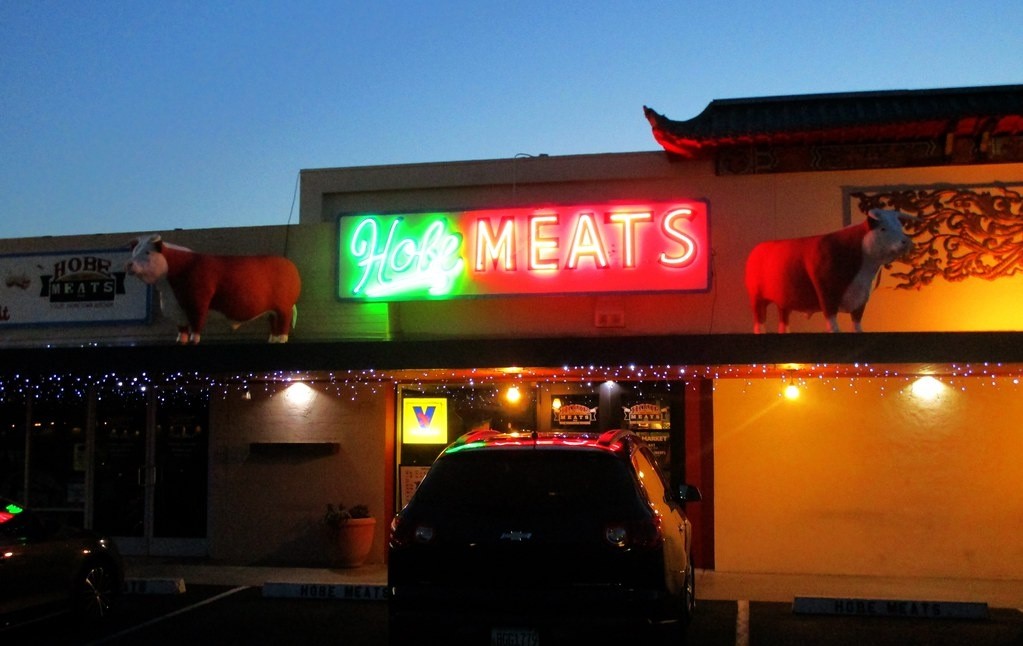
[[122, 235, 302, 345], [744, 208, 923, 333]]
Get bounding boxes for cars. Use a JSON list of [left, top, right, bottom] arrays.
[[0, 496, 121, 646]]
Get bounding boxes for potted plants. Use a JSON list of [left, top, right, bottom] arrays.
[[322, 502, 376, 568]]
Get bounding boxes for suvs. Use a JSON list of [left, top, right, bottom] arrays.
[[384, 426, 704, 646]]
